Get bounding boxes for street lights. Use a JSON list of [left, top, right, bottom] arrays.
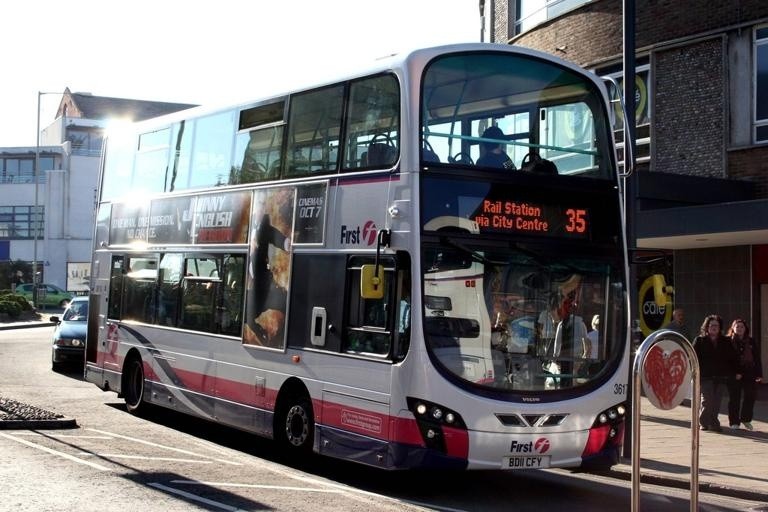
[[33, 91, 92, 309]]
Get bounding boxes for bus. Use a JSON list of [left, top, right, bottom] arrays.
[[83, 43, 630, 475]]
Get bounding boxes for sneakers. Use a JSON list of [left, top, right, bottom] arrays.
[[699, 415, 720, 432], [731, 421, 753, 430]]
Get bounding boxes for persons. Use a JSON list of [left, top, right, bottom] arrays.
[[691, 313, 729, 433], [476, 128, 519, 171], [490, 291, 644, 396], [248, 186, 291, 320], [725, 319, 763, 435], [663, 307, 690, 341]]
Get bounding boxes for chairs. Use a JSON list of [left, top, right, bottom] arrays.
[[230, 132, 559, 184]]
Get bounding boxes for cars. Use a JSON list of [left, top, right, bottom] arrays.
[[16, 283, 73, 309], [51, 296, 89, 370]]
